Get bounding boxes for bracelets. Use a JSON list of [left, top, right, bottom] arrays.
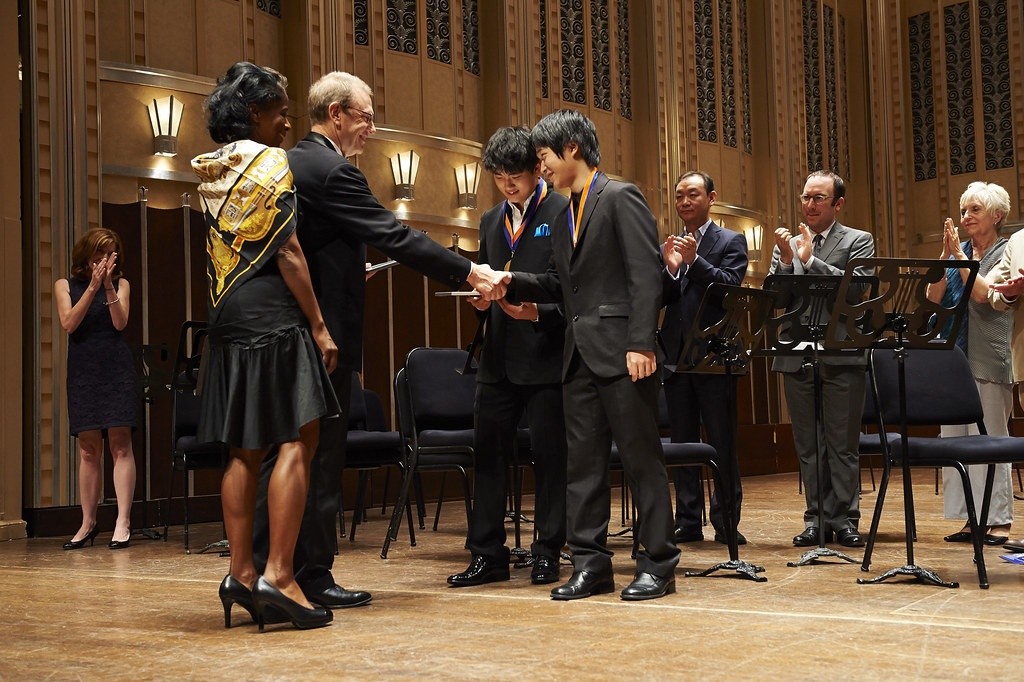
[[105, 287, 115, 290], [105, 297, 119, 305]]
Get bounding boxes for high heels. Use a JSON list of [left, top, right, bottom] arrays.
[[63, 522, 99, 550], [219, 574, 293, 629], [252, 574, 334, 632], [108, 523, 133, 549]]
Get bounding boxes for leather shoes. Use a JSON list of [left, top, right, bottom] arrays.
[[531, 555, 560, 583], [837, 527, 866, 547], [550, 568, 616, 600], [447, 554, 511, 585], [793, 526, 834, 545], [620, 570, 676, 600], [306, 583, 374, 608]]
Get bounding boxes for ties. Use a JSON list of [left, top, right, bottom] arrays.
[[812, 235, 823, 258]]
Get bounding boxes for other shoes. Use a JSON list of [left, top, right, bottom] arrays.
[[983, 523, 1012, 546], [715, 529, 748, 545], [672, 525, 704, 543], [943, 523, 974, 542]]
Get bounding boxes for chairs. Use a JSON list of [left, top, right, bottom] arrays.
[[799, 369, 901, 492], [862, 339, 1024, 589], [164, 348, 729, 560]]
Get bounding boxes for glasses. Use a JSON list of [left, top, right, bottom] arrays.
[[799, 195, 835, 205], [344, 105, 374, 124]]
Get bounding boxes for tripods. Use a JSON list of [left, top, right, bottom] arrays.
[[685, 256, 979, 588]]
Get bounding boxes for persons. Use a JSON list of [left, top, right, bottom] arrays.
[[447, 123, 566, 586], [53, 228, 143, 550], [762, 170, 878, 546], [656, 170, 748, 546], [252, 69, 509, 610], [492, 109, 682, 600], [925, 180, 1015, 545], [989, 227, 1024, 553], [191, 62, 332, 634]]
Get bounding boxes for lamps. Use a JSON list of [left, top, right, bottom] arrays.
[[146, 94, 185, 158], [742, 225, 764, 262], [453, 162, 482, 210], [390, 149, 421, 202]]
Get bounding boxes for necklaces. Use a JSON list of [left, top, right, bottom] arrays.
[[978, 236, 999, 262]]
